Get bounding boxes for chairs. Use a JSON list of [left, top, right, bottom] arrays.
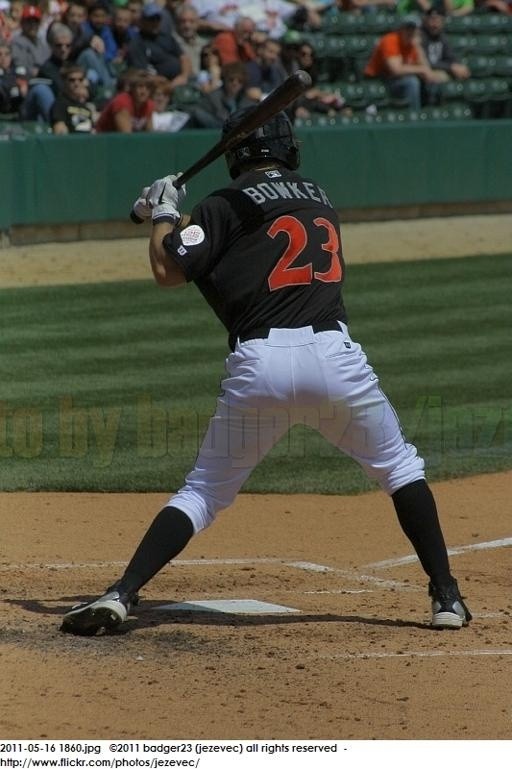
[[1, 0, 511, 136]]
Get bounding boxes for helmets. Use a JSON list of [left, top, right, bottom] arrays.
[[222, 104, 300, 180]]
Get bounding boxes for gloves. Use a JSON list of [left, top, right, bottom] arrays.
[[134, 172, 187, 226]]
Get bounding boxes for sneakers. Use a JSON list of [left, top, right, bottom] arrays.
[[427, 581, 471, 628], [60, 580, 137, 636]]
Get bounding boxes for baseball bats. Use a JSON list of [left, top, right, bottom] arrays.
[[129, 64, 315, 230]]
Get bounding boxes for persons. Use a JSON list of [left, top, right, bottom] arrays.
[[0, 1, 511, 133], [55, 99, 482, 636]]
